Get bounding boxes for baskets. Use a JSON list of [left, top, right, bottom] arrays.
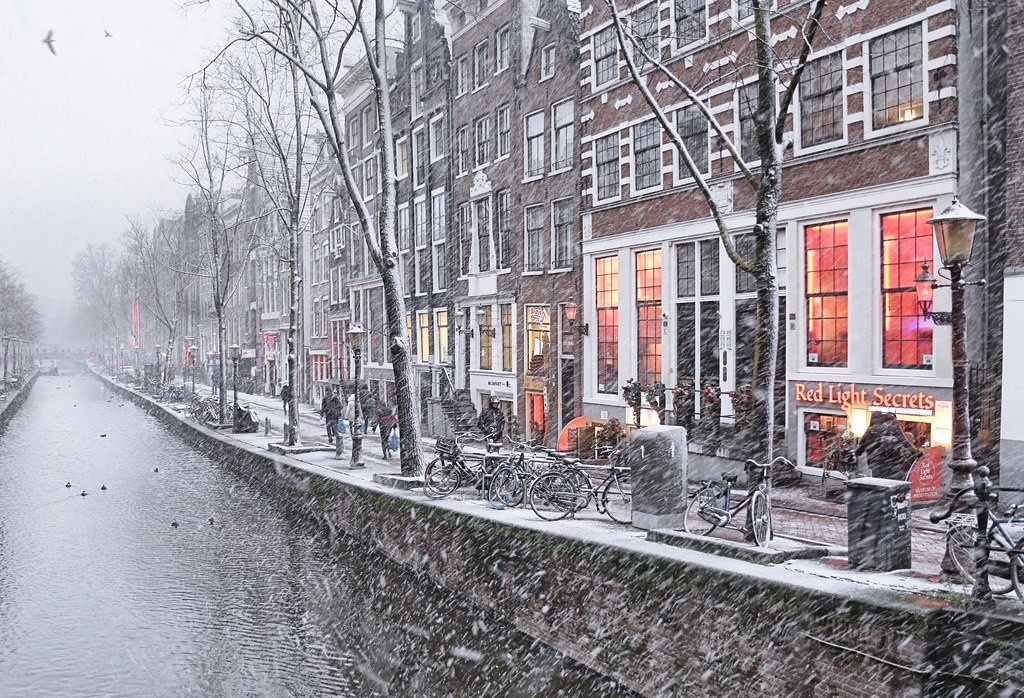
[[435, 436, 454, 454]]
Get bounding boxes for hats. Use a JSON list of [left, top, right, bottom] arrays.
[[489, 396, 500, 403]]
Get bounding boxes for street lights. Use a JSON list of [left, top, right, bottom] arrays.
[[229, 342, 242, 432], [11, 336, 18, 374], [2, 335, 10, 387], [19, 338, 24, 366], [133, 345, 140, 366], [912, 191, 985, 515], [190, 343, 198, 394], [154, 344, 162, 372], [346, 323, 367, 470]]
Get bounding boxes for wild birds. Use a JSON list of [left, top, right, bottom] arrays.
[[154, 468, 158, 472], [81, 491, 88, 496], [65, 482, 71, 487], [100, 434, 106, 438], [101, 484, 107, 490], [208, 517, 214, 525], [171, 521, 179, 528]]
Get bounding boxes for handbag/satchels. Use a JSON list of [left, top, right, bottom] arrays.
[[389, 428, 398, 452]]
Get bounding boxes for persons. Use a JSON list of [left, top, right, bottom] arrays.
[[318, 388, 343, 444], [478, 396, 505, 455], [210, 370, 219, 396], [346, 385, 397, 460], [851, 411, 924, 482], [280, 382, 290, 416]]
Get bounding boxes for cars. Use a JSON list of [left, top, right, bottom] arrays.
[[120, 366, 135, 374]]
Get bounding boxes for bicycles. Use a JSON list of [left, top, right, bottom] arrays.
[[927, 484, 1024, 605], [123, 372, 250, 425], [680, 454, 797, 549], [419, 429, 633, 525]]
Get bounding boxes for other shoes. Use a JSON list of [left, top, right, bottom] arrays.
[[382, 458, 387, 460], [388, 454, 392, 458]]
